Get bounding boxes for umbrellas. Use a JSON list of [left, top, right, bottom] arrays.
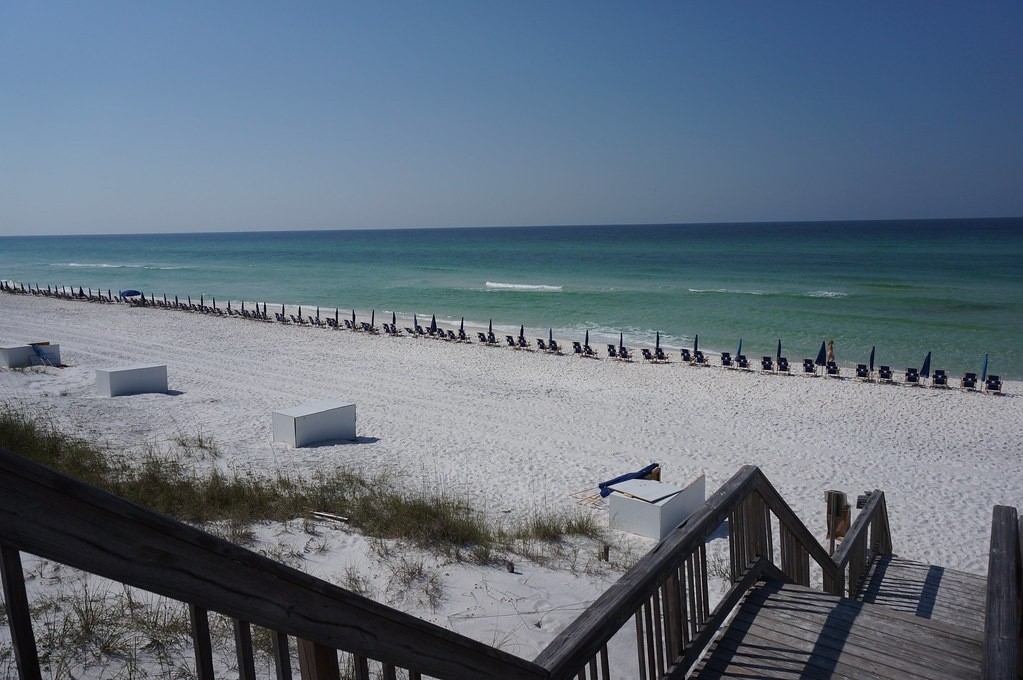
[[815, 341, 826, 376], [488, 318, 492, 344], [656, 331, 659, 358], [0, 280, 437, 336], [693, 334, 697, 359], [548, 328, 552, 350], [461, 317, 463, 341], [735, 338, 742, 367], [980, 353, 988, 391], [585, 330, 588, 357], [777, 339, 781, 371], [920, 351, 930, 387], [519, 324, 523, 345], [870, 346, 875, 381], [619, 332, 622, 360]]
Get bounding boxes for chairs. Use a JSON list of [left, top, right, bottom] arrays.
[[693, 351, 709, 368], [680, 349, 694, 366], [825, 361, 840, 378], [904, 368, 920, 388], [720, 353, 735, 370], [0, 281, 583, 357], [583, 345, 597, 359], [761, 356, 775, 375], [878, 365, 893, 384], [606, 345, 620, 360], [802, 359, 818, 377], [776, 357, 792, 376], [736, 355, 752, 371], [933, 369, 947, 390], [984, 374, 1003, 396], [855, 364, 871, 381], [618, 347, 632, 362], [654, 348, 670, 364], [959, 372, 978, 392], [641, 348, 658, 363]]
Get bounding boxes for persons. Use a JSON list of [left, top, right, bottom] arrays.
[[827, 340, 835, 367]]
